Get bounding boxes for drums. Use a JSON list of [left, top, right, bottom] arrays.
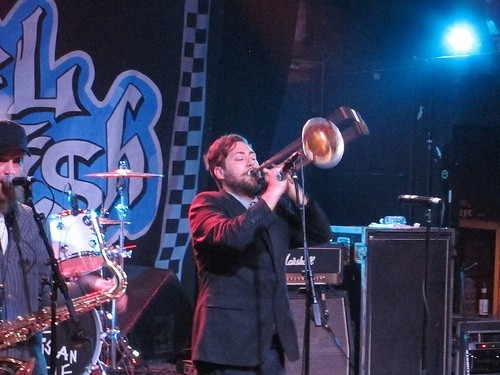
[[48, 209, 104, 277], [42, 308, 103, 375]]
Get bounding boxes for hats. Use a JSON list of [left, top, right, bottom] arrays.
[[0, 120, 31, 157]]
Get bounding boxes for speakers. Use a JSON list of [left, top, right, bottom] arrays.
[[283, 291, 355, 375], [367, 232, 449, 375], [116, 265, 197, 361]]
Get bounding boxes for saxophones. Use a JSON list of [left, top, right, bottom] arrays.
[[0, 244, 127, 375]]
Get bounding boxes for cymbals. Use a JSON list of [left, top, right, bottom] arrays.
[[82, 168, 165, 178], [98, 218, 132, 226]]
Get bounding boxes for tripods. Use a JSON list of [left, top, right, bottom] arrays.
[[102, 300, 154, 375]]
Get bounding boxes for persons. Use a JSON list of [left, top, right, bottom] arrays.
[[188, 134, 332, 375], [0, 118, 129, 375]]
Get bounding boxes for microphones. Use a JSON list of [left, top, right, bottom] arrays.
[[320, 317, 330, 329], [399, 194, 443, 205], [274, 153, 300, 181], [67, 184, 73, 202], [317, 286, 329, 317], [70, 329, 94, 343], [2, 175, 37, 188]]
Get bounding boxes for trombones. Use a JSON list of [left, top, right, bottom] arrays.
[[247, 107, 370, 183]]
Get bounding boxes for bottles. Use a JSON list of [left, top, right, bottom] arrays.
[[379, 216, 407, 225], [478, 282, 489, 318]]
